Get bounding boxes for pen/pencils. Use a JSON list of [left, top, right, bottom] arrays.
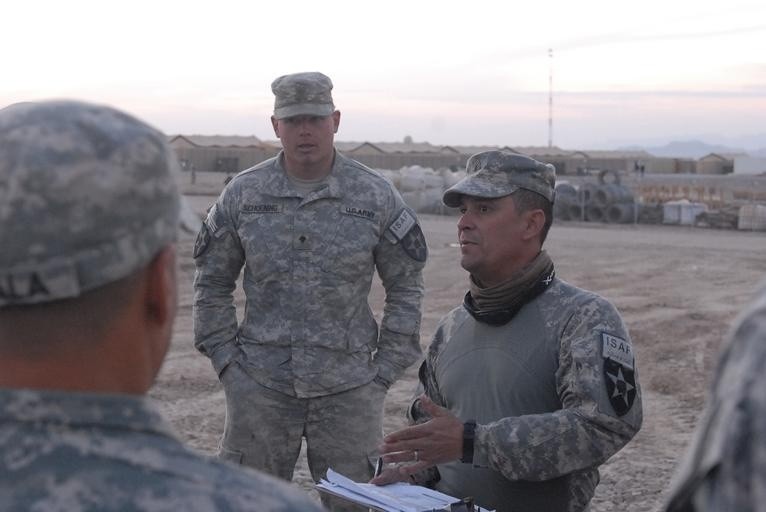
[[368, 458, 382, 512]]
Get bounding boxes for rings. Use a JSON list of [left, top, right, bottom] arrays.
[[414, 450, 419, 461]]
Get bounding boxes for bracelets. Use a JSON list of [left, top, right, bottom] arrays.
[[459, 421, 476, 464]]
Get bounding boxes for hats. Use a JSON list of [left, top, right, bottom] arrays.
[[0, 100, 204, 308], [443, 149, 557, 208]]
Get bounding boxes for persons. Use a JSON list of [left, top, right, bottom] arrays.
[[1, 99, 326, 512], [364, 151, 645, 512], [192, 72, 429, 490]]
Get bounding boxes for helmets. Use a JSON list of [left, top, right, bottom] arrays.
[[271, 71, 336, 122]]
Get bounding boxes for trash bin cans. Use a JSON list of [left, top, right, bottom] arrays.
[[662, 202, 706, 225]]
